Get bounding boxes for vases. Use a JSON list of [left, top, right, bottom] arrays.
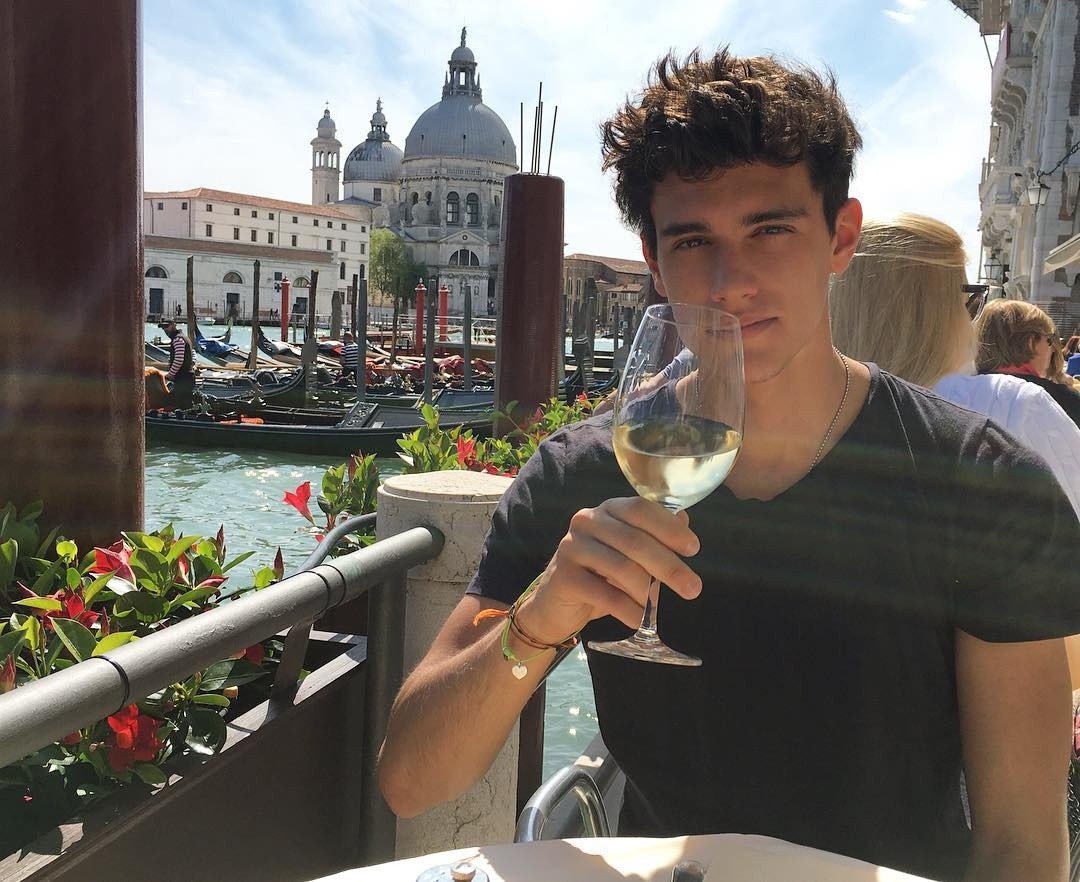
[[1, 603, 375, 881]]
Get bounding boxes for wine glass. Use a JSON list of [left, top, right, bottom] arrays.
[[588, 305, 747, 666]]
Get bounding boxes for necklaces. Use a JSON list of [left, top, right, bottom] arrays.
[[695, 346, 850, 472]]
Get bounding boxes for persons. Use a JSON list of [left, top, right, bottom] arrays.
[[158, 318, 195, 409], [375, 49, 1080, 882], [826, 211, 1080, 691], [340, 332, 358, 384], [1041, 331, 1080, 390], [973, 297, 1080, 430], [269, 308, 278, 320]]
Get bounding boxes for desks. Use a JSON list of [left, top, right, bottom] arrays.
[[306, 834, 937, 882]]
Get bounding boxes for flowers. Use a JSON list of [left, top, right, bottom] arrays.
[[288, 393, 611, 557], [1, 501, 292, 834]]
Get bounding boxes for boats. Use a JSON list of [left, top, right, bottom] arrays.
[[142, 255, 622, 458]]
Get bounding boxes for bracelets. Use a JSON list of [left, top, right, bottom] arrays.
[[473, 571, 585, 679]]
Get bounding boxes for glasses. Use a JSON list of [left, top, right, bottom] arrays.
[[962, 285, 989, 324], [671, 859, 705, 882], [1047, 335, 1054, 346], [162, 325, 169, 329]]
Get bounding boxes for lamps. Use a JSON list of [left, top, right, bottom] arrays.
[[1028, 140, 1080, 207], [1053, 266, 1080, 290], [983, 253, 1002, 279]]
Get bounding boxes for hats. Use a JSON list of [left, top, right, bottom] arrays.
[[158, 316, 175, 328]]
